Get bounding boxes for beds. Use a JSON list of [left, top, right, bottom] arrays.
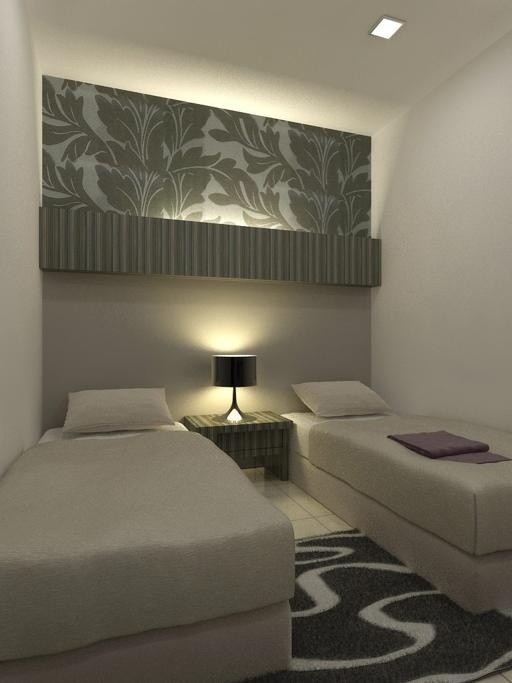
[[281, 412, 512, 615], [0, 421, 294, 683]]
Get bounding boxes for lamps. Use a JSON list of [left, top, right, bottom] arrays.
[[211, 355, 256, 424]]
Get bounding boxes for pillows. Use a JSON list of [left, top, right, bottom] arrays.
[[291, 381, 393, 416], [63, 388, 173, 433]]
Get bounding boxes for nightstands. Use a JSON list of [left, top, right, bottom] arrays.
[[185, 412, 293, 481]]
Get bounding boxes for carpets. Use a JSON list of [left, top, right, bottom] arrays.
[[288, 529, 512, 683]]
